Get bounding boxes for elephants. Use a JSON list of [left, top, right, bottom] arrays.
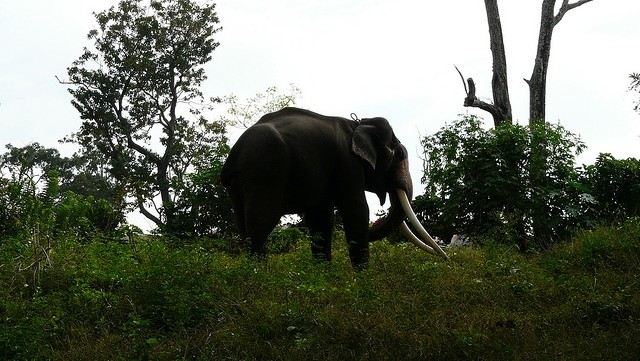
[[220, 106, 449, 274]]
